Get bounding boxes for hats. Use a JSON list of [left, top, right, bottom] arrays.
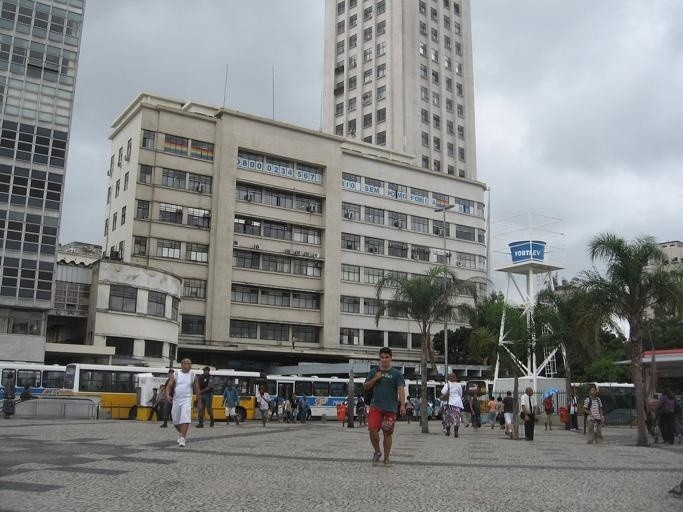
[[202, 367, 210, 372]]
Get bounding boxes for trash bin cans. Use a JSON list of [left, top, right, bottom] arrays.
[[560, 406, 568, 423], [337, 404, 347, 420]]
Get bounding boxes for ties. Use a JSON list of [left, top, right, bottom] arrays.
[[529, 396, 532, 413]]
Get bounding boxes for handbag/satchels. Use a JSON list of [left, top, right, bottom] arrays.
[[3, 398, 16, 416], [520, 411, 529, 421], [437, 382, 451, 401], [164, 372, 178, 397], [363, 368, 382, 405]]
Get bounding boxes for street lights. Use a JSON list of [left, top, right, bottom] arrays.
[[434, 204, 454, 384]]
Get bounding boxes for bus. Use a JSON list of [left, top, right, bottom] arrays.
[[397, 379, 467, 420], [62, 362, 267, 423], [570, 381, 635, 392], [264, 374, 366, 421], [0, 361, 66, 415], [464, 379, 494, 416]]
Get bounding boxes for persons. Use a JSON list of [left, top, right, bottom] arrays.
[[2, 371, 16, 419], [360, 346, 406, 466], [17, 382, 41, 403], [163, 356, 200, 447]]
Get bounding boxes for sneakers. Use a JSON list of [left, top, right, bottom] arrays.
[[570, 427, 579, 431], [195, 420, 240, 428], [176, 433, 185, 446], [504, 431, 534, 441], [667, 480, 683, 498], [359, 422, 368, 426], [259, 417, 312, 426], [446, 430, 459, 438], [587, 439, 600, 445], [159, 423, 168, 428], [4, 415, 10, 419], [654, 436, 675, 445], [371, 451, 392, 468], [545, 428, 552, 431], [465, 424, 505, 432]]
